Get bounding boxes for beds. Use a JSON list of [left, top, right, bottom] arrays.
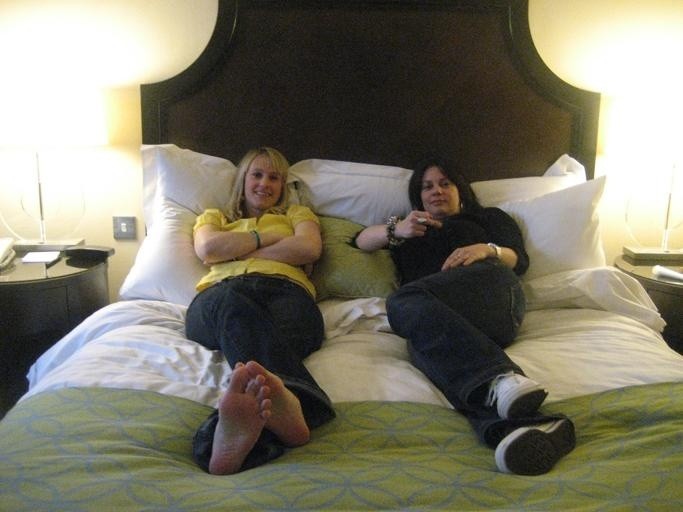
[[0, 2, 682, 511]]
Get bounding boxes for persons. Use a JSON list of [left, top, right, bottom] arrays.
[[352, 157, 575, 476], [186, 145, 336, 474]]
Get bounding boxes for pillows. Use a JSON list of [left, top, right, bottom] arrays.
[[304, 213, 400, 304], [457, 154, 585, 212], [117, 146, 307, 307], [498, 171, 605, 287], [280, 154, 416, 229]]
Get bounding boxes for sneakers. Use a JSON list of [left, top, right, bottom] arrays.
[[496, 373, 549, 419], [495, 419, 576, 475]]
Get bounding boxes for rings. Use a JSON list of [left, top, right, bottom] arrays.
[[417, 216, 427, 224]]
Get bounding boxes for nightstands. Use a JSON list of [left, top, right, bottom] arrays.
[[0, 234, 111, 420], [614, 251, 683, 356]]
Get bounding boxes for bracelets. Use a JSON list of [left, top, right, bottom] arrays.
[[251, 230, 261, 250], [386, 216, 403, 246], [488, 242, 502, 260], [232, 257, 239, 261]]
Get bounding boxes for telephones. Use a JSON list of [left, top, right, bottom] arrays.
[[0, 237, 15, 269]]
